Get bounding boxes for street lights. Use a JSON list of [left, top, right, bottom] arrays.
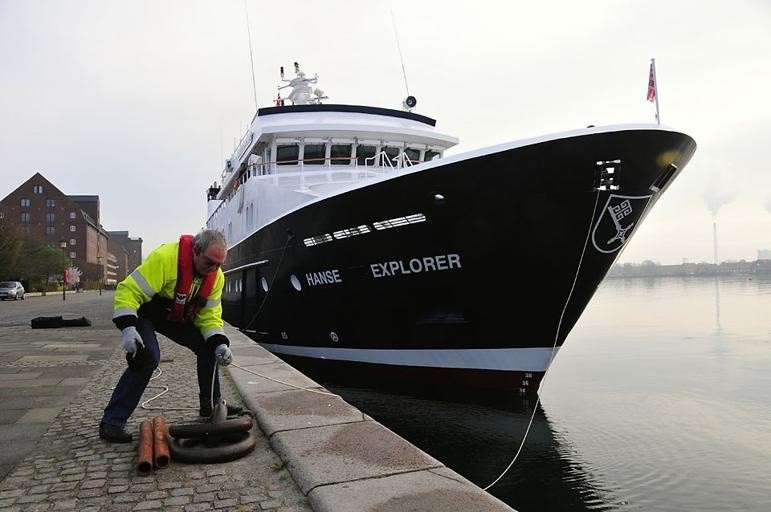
[[58, 235, 69, 301], [96, 255, 105, 296]]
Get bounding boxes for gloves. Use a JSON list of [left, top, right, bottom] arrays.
[[121, 326, 146, 358], [214, 344, 234, 367]]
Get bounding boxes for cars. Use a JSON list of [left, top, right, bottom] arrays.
[[0, 281, 26, 301]]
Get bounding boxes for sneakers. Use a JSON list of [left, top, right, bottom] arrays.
[[199, 397, 243, 417], [99, 423, 133, 444]]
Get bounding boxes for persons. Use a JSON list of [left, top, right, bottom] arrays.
[[100, 228, 244, 442]]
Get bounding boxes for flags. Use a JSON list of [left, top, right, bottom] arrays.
[[646, 62, 656, 102]]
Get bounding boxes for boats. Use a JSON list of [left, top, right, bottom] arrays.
[[197, 61, 699, 417]]
